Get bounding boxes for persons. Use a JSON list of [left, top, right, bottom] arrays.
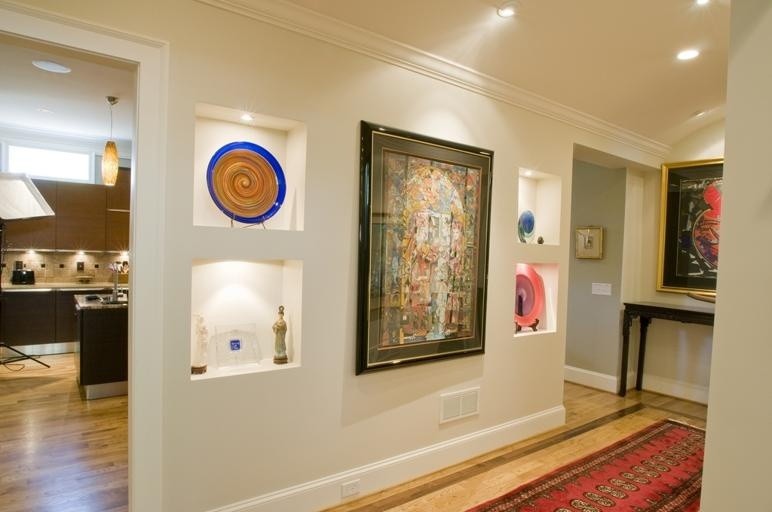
[[272, 305, 288, 358], [194, 312, 210, 366]]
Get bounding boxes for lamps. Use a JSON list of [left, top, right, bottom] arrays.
[[100, 96, 119, 185]]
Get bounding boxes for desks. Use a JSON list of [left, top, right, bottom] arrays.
[[617, 298, 714, 399]]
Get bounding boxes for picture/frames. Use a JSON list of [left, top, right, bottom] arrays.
[[352, 119, 496, 378], [573, 225, 604, 261], [652, 154, 726, 301]]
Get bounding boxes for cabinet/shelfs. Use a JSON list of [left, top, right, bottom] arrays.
[[0, 180, 104, 252], [103, 167, 131, 253], [0, 290, 104, 347]]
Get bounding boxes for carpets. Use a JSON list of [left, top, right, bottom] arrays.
[[461, 417, 706, 512]]
